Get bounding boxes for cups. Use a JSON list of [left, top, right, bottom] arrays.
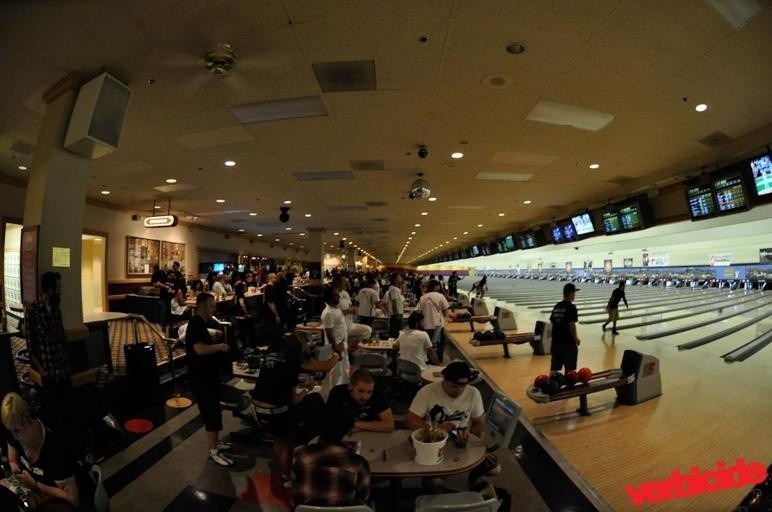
[[389, 337, 394, 346]]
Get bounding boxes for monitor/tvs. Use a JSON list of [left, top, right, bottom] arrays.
[[751, 154, 772, 197], [602, 210, 621, 236], [686, 183, 716, 223], [621, 203, 647, 230], [214, 264, 225, 272], [552, 225, 564, 243], [563, 222, 575, 240], [714, 179, 749, 212], [571, 213, 595, 236], [443, 234, 542, 262]]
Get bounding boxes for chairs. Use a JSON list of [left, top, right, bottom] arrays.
[[121, 275, 520, 512]]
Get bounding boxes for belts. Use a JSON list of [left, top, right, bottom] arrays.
[[251, 402, 288, 415]]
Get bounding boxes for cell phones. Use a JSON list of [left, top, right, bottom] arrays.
[[451, 427, 461, 437]]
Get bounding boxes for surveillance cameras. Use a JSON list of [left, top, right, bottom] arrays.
[[417, 148, 428, 158]]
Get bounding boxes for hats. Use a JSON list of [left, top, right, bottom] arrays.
[[564, 284, 580, 292], [442, 361, 471, 385]]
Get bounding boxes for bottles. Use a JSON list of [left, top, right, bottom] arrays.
[[0, 462, 36, 510], [303, 316, 307, 326]]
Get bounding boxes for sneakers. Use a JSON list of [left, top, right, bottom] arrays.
[[209, 453, 234, 467], [215, 440, 232, 450]]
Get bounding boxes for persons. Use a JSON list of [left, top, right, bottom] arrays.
[[0, 250, 495, 511], [547, 283, 583, 377], [601, 278, 628, 335]]
